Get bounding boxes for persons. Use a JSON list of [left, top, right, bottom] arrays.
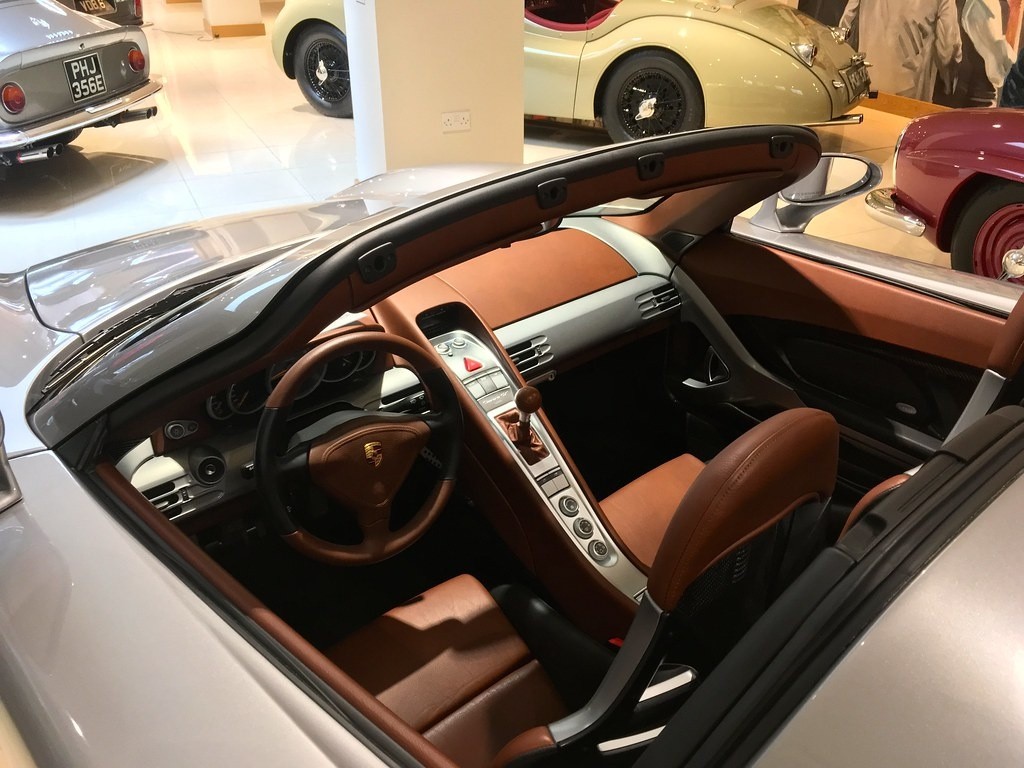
[[932, 0, 1017, 110], [838, 0, 962, 105]]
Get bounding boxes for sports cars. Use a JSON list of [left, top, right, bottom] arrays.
[[1, 123, 1024, 768]]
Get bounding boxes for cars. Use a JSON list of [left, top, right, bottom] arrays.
[[268, 0, 878, 146], [3, 0, 160, 159], [862, 109, 1024, 287], [58, 0, 142, 30]]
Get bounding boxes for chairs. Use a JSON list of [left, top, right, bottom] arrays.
[[319, 405, 840, 768], [597, 292, 1024, 569]]
